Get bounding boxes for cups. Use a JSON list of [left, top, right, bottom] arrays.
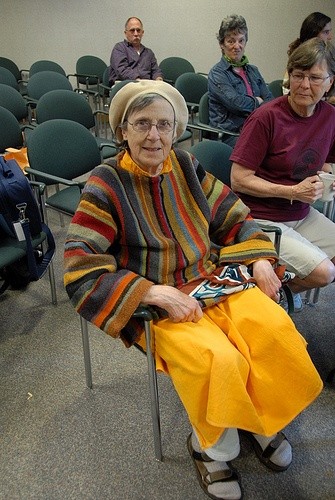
[[318, 173, 335, 202]]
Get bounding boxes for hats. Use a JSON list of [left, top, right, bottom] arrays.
[[109, 79, 189, 145]]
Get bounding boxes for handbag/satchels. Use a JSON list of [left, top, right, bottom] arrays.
[[0, 155, 42, 242]]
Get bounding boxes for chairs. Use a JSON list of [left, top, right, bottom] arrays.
[[0, 55, 335, 463]]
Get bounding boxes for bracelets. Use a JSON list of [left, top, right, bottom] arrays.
[[290, 186, 294, 205]]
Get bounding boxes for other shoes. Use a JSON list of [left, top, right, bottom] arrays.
[[281, 282, 302, 312]]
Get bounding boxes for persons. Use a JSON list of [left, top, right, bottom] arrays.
[[231, 38, 335, 314], [106, 17, 163, 84], [208, 15, 274, 149], [63, 79, 324, 500], [283, 11, 335, 105]]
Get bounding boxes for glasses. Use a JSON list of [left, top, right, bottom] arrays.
[[124, 118, 176, 134], [289, 72, 333, 84]]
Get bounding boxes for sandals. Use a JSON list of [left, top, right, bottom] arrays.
[[239, 428, 293, 472], [187, 433, 245, 500]]
